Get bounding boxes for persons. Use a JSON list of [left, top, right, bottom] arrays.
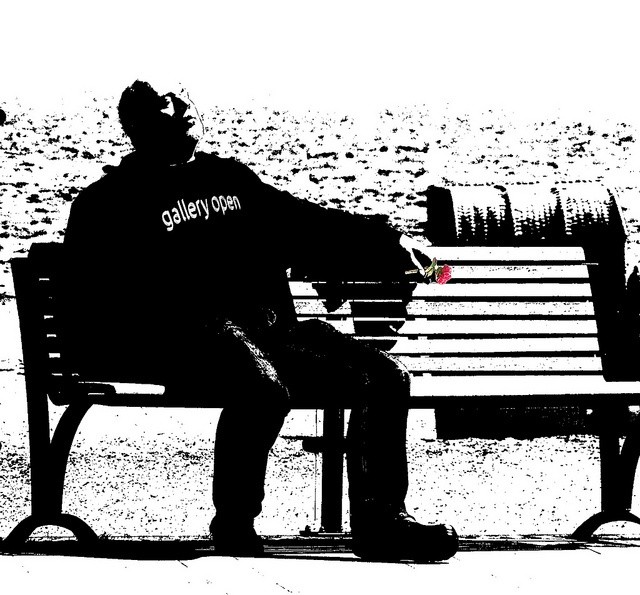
[[51, 77, 463, 561]]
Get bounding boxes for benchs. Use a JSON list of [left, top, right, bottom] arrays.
[[1, 239, 640, 552]]
